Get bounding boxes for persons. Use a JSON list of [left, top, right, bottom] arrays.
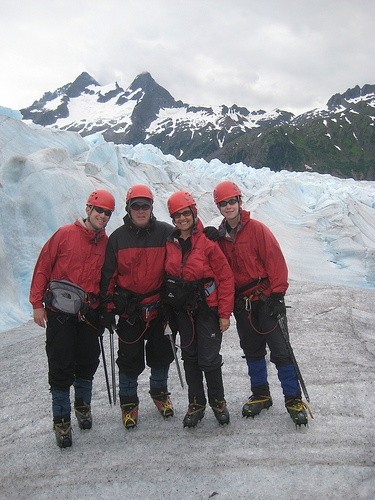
[[163, 191, 234, 429], [99, 184, 221, 430], [30, 190, 115, 448], [213, 182, 314, 430]]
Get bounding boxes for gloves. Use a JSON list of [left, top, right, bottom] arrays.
[[98, 312, 114, 335], [268, 293, 285, 319]]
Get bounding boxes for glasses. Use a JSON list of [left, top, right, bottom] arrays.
[[173, 210, 193, 218], [90, 206, 113, 216], [129, 202, 152, 211], [219, 199, 239, 207]]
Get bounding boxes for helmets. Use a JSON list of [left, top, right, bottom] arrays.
[[86, 190, 115, 211], [214, 181, 241, 204], [167, 191, 196, 217], [126, 184, 154, 203]]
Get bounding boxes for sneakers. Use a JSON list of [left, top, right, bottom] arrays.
[[74, 402, 92, 430], [151, 392, 174, 417], [119, 396, 139, 430], [183, 398, 207, 428], [242, 392, 274, 419], [53, 412, 72, 448], [209, 398, 230, 426], [283, 396, 308, 427]]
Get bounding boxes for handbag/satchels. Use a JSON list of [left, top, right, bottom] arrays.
[[112, 286, 140, 314], [163, 276, 198, 310], [45, 280, 84, 317]]
